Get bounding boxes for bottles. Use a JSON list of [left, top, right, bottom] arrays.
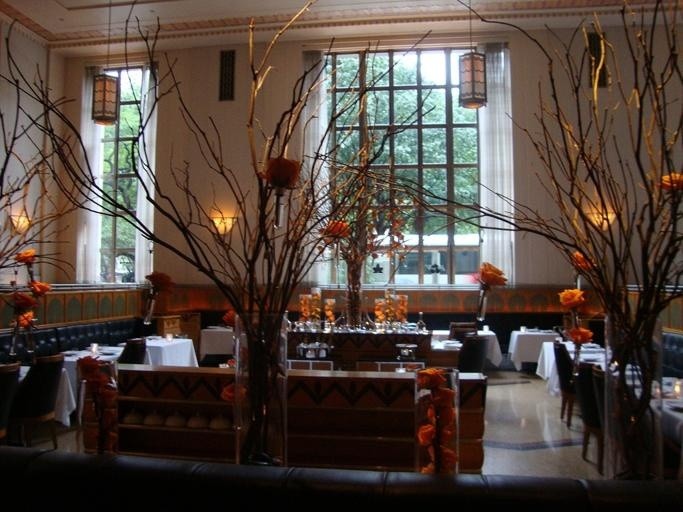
[[417, 311, 427, 332]]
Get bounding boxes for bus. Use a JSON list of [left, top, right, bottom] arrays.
[[365, 233, 480, 287]]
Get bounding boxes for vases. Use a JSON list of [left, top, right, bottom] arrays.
[[602, 314, 665, 479], [144, 298, 157, 325], [233, 311, 289, 467], [476, 289, 488, 321]]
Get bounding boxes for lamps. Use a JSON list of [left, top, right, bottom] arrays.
[[92, 0, 120, 126], [458, 0, 488, 110]]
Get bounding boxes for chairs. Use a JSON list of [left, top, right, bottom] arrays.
[[409, 322, 496, 377], [542, 341, 683, 476], [0, 337, 147, 449]]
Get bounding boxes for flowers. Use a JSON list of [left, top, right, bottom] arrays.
[[556, 244, 593, 385], [413, 363, 458, 475], [221, 383, 248, 430], [72, 351, 121, 455], [257, 154, 352, 243], [475, 262, 507, 317], [144, 269, 177, 319], [11, 247, 53, 331]]
